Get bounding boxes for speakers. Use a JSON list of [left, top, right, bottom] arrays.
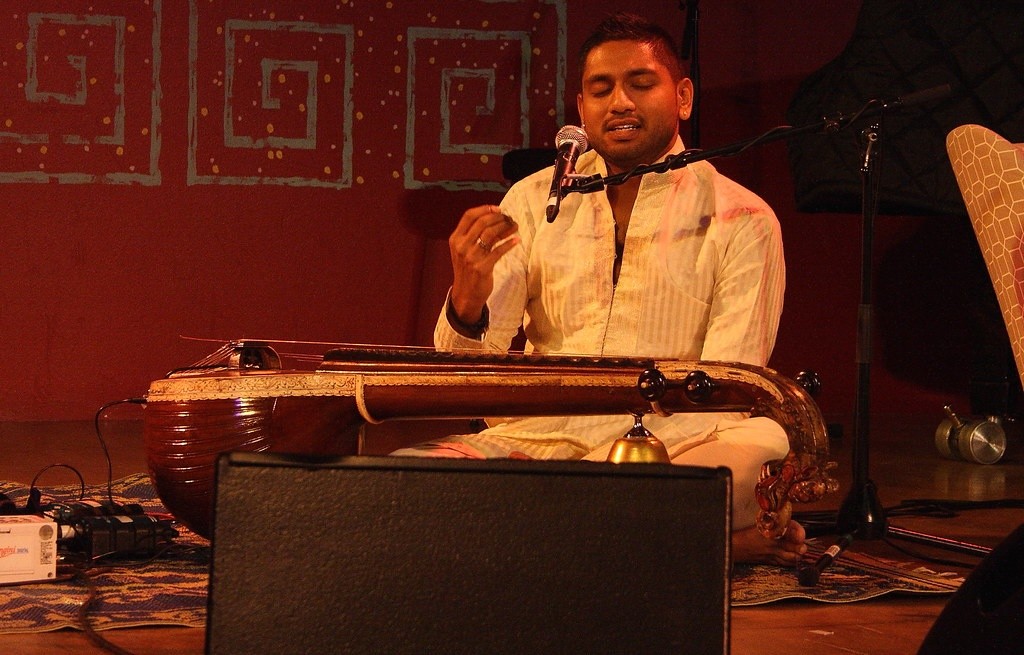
[[202, 448, 733, 655]]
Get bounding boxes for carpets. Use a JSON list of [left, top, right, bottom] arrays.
[[1, 471, 973, 636]]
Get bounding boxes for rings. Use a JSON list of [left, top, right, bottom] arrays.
[[476, 237, 491, 250]]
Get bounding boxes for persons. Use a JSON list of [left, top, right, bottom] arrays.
[[916, 122, 1024, 655], [388, 12, 807, 570]]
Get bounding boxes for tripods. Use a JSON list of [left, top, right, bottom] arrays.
[[790, 121, 998, 589]]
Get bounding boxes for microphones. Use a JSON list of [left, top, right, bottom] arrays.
[[545, 123, 590, 224]]
